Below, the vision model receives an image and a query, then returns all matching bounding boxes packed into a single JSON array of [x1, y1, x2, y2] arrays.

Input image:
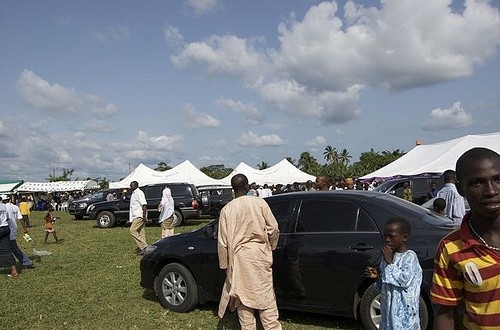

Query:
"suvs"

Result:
[[67, 188, 122, 219], [196, 186, 235, 217], [86, 183, 210, 229], [373, 175, 451, 206]]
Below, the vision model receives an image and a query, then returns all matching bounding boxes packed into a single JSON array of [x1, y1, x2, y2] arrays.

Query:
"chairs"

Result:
[[50, 202, 70, 211]]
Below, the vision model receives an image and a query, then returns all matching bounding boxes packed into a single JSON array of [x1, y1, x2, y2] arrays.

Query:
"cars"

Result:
[[139, 191, 464, 330]]
